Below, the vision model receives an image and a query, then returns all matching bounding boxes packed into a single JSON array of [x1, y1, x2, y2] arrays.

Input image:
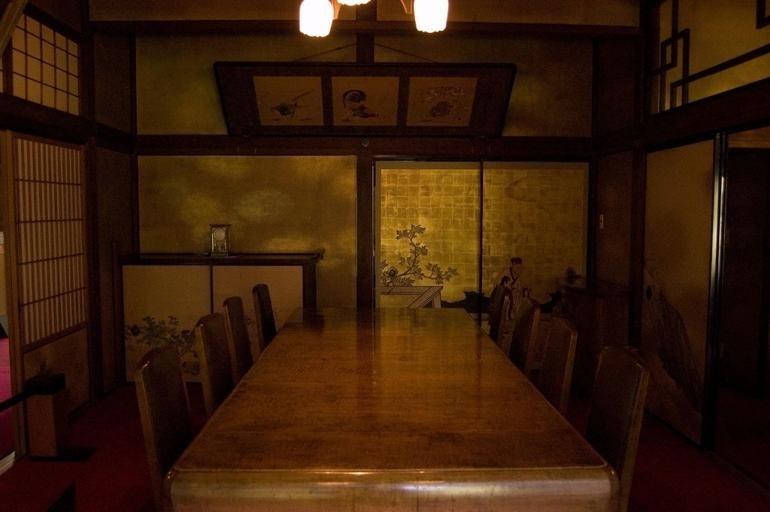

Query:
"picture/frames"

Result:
[[211, 59, 517, 139]]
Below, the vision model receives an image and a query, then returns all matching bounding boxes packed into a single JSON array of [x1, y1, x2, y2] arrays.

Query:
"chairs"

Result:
[[537, 316, 579, 416], [581, 343, 650, 511], [222, 295, 253, 388], [194, 312, 233, 423], [253, 283, 276, 353], [508, 297, 542, 379], [137, 340, 195, 512], [488, 285, 521, 360]]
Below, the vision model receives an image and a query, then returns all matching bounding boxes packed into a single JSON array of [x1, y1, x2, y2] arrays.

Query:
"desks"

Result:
[[164, 307, 622, 511]]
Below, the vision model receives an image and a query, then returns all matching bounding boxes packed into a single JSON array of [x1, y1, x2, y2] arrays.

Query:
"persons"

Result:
[[488, 257, 561, 328]]
